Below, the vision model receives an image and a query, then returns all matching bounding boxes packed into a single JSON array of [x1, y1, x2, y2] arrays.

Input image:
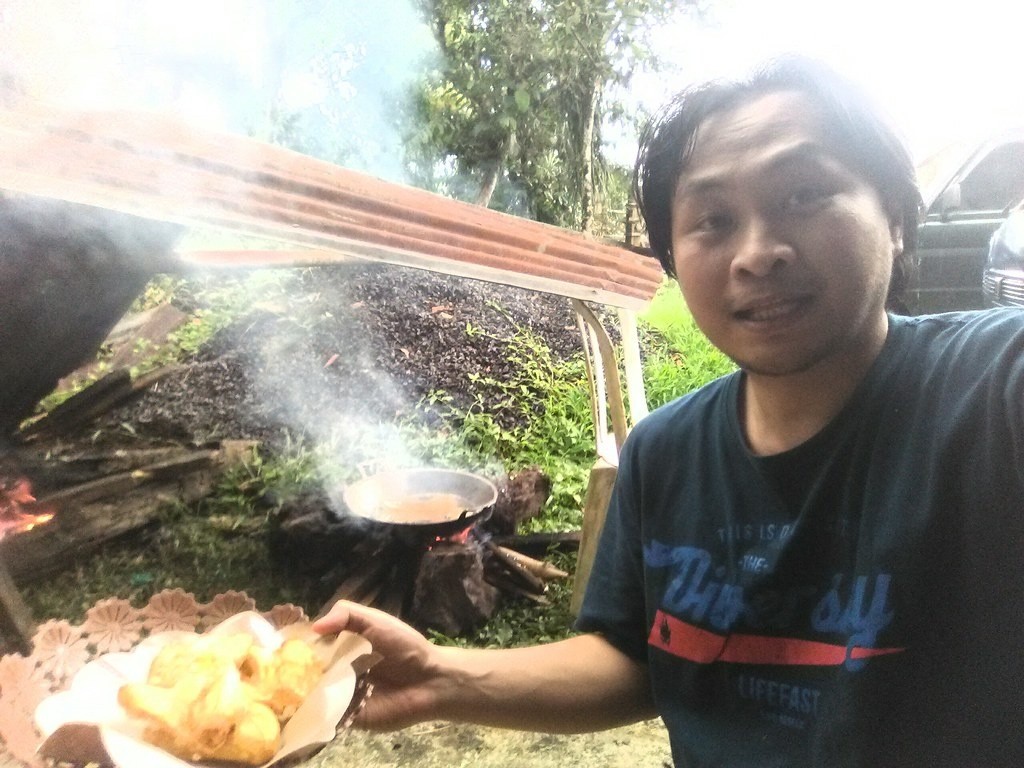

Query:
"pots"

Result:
[[344, 467, 499, 538]]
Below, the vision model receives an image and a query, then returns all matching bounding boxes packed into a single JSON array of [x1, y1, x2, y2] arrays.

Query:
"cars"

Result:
[[880, 113, 1023, 316]]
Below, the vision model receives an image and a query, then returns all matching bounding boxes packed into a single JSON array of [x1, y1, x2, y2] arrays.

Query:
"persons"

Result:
[[301, 62, 1024, 767]]
